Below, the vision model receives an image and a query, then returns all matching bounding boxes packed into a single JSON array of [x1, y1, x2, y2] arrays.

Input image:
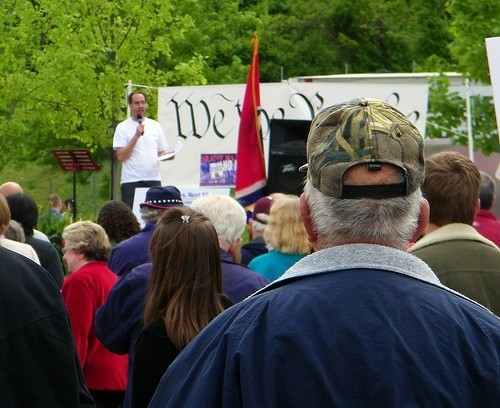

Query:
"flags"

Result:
[[234, 37, 267, 236]]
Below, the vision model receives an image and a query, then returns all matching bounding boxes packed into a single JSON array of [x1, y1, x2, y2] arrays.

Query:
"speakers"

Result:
[[267, 119, 312, 196]]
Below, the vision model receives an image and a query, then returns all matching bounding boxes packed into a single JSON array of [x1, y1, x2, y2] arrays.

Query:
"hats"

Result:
[[299, 97, 424, 199], [140, 186, 183, 209], [253, 196, 274, 225]]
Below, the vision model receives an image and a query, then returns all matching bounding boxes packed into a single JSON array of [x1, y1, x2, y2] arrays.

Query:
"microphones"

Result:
[[137, 114, 143, 136]]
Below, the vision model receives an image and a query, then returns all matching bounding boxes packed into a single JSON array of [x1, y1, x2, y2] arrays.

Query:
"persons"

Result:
[[113, 90, 176, 211], [0, 97, 500, 408]]
